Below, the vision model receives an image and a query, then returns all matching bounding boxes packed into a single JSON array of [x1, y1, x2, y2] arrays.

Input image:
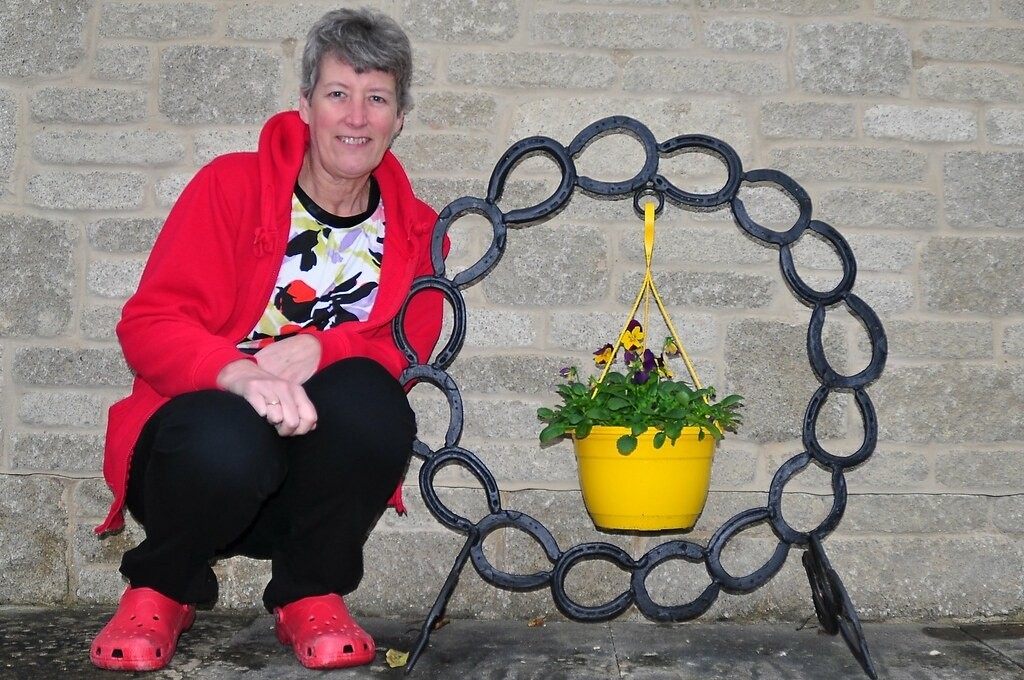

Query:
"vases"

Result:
[[564, 425, 723, 530]]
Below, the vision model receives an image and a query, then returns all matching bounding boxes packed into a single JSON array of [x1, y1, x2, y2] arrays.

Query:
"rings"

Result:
[[267, 401, 279, 406]]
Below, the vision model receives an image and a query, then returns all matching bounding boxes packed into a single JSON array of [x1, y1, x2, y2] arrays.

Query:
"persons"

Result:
[[90, 9, 449, 669]]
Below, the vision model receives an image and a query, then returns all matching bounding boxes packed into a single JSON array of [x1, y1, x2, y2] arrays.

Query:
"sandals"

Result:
[[88, 588, 197, 669], [273, 592, 376, 668]]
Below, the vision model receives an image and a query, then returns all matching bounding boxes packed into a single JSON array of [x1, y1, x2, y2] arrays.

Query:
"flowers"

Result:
[[537, 320, 745, 455]]
